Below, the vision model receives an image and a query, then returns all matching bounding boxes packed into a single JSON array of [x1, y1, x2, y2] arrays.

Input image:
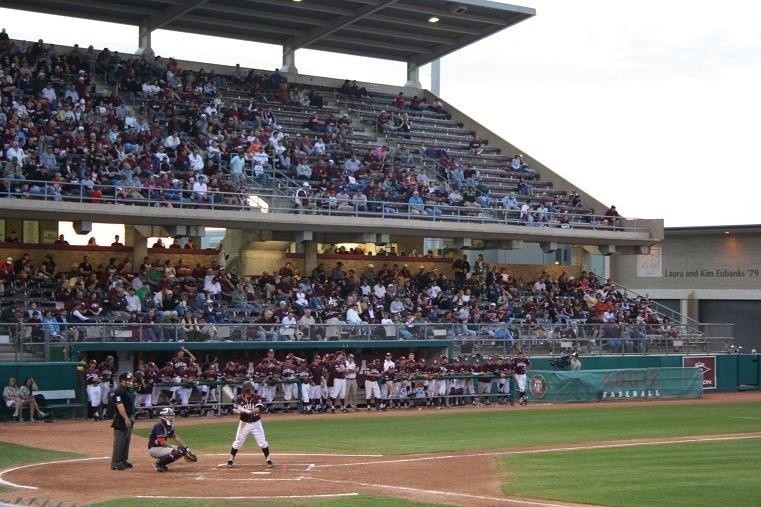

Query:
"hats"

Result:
[[571, 351, 579, 356], [147, 345, 517, 377], [89, 358, 98, 363]]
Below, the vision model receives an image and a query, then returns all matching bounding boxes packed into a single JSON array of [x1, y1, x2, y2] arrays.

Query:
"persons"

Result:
[[492, 352, 530, 405], [148, 407, 194, 474], [496, 152, 624, 232], [231, 63, 490, 222], [0, 230, 238, 342], [751, 349, 759, 355], [109, 368, 136, 470], [1, 27, 249, 211], [492, 263, 676, 353], [278, 352, 359, 414], [132, 348, 284, 415], [571, 352, 582, 370], [86, 355, 118, 420], [734, 346, 743, 355], [364, 352, 491, 411], [240, 243, 491, 341], [216, 379, 277, 469], [3, 375, 49, 423]]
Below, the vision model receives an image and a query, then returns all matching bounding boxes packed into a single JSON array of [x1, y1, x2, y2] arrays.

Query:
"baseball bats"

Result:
[[223, 385, 242, 410]]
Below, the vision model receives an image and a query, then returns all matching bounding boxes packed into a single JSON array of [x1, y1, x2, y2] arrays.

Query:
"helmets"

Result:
[[119, 370, 133, 382], [239, 380, 256, 394], [159, 407, 175, 416]]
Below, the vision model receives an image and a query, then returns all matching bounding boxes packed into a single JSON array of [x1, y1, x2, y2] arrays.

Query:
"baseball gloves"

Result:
[[184, 447, 197, 462]]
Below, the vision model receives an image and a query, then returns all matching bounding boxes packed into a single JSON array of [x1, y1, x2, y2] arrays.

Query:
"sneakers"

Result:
[[227, 454, 234, 464], [112, 461, 133, 469], [265, 454, 273, 464], [264, 393, 527, 414], [152, 458, 169, 471], [12, 410, 110, 421]]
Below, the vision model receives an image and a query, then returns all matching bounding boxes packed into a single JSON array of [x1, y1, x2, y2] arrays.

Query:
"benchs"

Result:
[[97, 70, 610, 230], [0, 272, 710, 353], [9, 389, 82, 422]]
